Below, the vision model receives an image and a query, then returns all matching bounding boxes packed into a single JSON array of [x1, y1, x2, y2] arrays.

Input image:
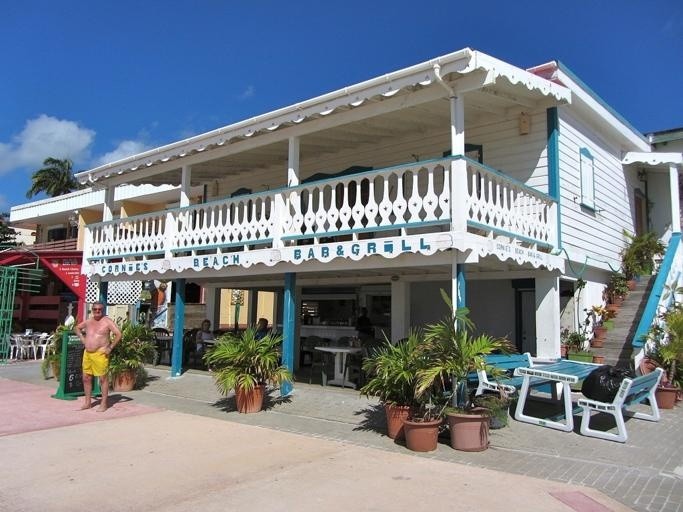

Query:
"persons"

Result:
[[254, 319, 268, 341], [196, 320, 216, 372], [74, 301, 122, 411], [303, 309, 313, 325], [355, 307, 371, 345]]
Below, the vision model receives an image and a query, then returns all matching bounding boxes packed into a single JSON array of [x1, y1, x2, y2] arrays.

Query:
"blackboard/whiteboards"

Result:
[[61, 331, 101, 397]]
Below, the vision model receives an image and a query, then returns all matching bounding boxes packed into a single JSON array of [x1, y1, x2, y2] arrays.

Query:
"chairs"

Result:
[[10, 328, 54, 362], [145, 324, 353, 369]]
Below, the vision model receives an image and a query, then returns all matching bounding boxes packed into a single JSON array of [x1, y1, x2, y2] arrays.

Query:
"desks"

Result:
[[313, 345, 363, 391], [510, 359, 605, 434]]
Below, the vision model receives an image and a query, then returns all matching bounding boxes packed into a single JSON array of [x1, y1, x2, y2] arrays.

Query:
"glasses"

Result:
[[94, 308, 101, 311]]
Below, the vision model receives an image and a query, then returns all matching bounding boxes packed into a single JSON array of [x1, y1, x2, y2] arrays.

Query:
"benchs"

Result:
[[470, 352, 559, 416], [575, 366, 665, 445]]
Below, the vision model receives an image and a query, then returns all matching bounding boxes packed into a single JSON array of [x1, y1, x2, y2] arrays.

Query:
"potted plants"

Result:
[[104, 316, 159, 393], [559, 229, 682, 410], [356, 286, 522, 453], [202, 324, 297, 415]]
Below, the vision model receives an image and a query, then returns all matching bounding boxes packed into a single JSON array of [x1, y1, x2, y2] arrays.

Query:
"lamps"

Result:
[[514, 111, 532, 136]]
